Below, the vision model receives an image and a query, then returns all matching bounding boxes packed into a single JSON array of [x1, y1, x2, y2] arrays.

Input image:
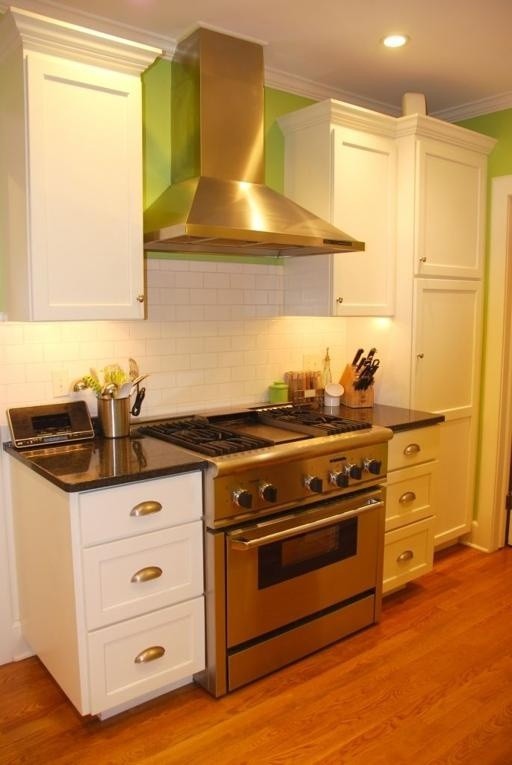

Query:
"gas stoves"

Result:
[[138, 404, 373, 457]]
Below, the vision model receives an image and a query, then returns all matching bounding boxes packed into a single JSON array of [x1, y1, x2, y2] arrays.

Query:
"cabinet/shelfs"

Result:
[[6, 455, 209, 723], [274, 96, 399, 318], [0, 5, 164, 322], [349, 112, 501, 554], [383, 423, 442, 599]]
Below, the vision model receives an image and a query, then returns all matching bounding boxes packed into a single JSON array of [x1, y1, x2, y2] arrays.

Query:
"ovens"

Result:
[[212, 443, 388, 694]]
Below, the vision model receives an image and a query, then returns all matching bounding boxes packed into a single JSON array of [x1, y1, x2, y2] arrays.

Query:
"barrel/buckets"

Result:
[[96, 394, 131, 438]]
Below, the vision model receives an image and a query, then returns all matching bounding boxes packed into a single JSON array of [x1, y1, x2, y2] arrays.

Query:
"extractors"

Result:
[[144, 27, 366, 259]]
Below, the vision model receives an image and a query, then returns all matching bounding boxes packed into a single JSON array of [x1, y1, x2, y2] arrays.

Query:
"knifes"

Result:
[[352, 348, 380, 391]]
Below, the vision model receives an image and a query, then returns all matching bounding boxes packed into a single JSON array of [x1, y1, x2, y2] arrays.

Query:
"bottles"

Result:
[[322, 347, 333, 388], [269, 379, 289, 404]]
